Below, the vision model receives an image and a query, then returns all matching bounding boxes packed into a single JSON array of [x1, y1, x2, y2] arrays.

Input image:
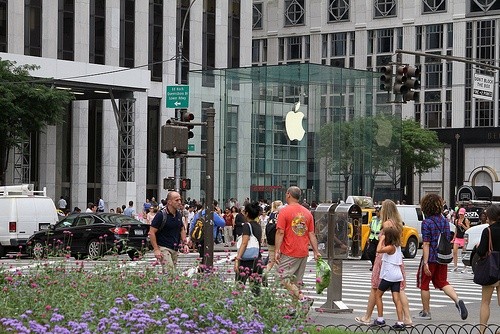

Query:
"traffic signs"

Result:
[[166, 85, 190, 109]]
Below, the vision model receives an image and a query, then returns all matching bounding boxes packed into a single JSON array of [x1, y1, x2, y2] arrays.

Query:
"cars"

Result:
[[27, 212, 151, 261], [461, 223, 490, 266], [348, 208, 420, 260]]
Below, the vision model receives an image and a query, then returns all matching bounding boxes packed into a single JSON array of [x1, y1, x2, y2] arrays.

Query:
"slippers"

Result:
[[355, 317, 372, 325], [404, 323, 414, 328]]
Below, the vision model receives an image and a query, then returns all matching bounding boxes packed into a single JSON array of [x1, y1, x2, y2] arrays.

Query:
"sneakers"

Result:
[[373, 319, 385, 327], [390, 322, 404, 331], [415, 310, 431, 320], [455, 299, 468, 320]]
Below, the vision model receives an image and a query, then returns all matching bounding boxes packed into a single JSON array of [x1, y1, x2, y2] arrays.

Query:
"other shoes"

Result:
[[461, 266, 469, 273], [286, 308, 296, 315], [370, 266, 373, 271], [299, 297, 314, 319], [450, 267, 458, 272]]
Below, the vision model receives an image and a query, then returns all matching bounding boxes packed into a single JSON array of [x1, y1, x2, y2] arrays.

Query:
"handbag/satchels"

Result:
[[237, 222, 259, 261], [474, 227, 500, 285], [316, 256, 332, 294]]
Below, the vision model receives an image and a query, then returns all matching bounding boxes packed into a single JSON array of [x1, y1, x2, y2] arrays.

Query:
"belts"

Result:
[[371, 231, 379, 234]]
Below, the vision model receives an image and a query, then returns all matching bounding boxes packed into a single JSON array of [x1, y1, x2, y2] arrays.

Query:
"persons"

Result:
[[354, 199, 415, 329], [149, 191, 190, 283], [273, 186, 322, 320], [233, 202, 263, 299], [412, 194, 470, 320], [470, 205, 500, 334], [56, 195, 500, 332], [370, 227, 407, 331]]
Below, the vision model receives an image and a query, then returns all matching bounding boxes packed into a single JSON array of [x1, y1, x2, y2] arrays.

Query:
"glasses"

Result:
[[375, 211, 379, 213]]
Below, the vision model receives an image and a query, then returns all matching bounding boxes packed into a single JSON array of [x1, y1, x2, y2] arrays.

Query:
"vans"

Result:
[[376, 205, 457, 249], [0, 184, 59, 257]]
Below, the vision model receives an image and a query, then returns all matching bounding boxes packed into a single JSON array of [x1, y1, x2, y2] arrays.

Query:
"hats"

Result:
[[374, 205, 382, 211], [459, 208, 465, 215]]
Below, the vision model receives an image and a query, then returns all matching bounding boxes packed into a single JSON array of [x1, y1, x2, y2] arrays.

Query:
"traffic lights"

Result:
[[380, 65, 392, 90], [404, 65, 420, 103], [180, 178, 191, 191], [164, 177, 174, 191], [395, 63, 409, 95], [181, 109, 195, 139]]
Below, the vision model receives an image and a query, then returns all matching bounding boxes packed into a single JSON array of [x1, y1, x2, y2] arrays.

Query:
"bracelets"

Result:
[[235, 256, 240, 261], [182, 244, 189, 249], [423, 262, 428, 265]]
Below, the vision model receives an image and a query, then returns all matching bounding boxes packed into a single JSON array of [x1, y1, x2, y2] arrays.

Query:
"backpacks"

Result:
[[266, 218, 276, 245], [190, 211, 204, 244], [430, 218, 453, 265]]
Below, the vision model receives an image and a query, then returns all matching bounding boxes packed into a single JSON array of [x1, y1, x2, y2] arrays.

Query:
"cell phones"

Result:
[[214, 207, 218, 211]]
